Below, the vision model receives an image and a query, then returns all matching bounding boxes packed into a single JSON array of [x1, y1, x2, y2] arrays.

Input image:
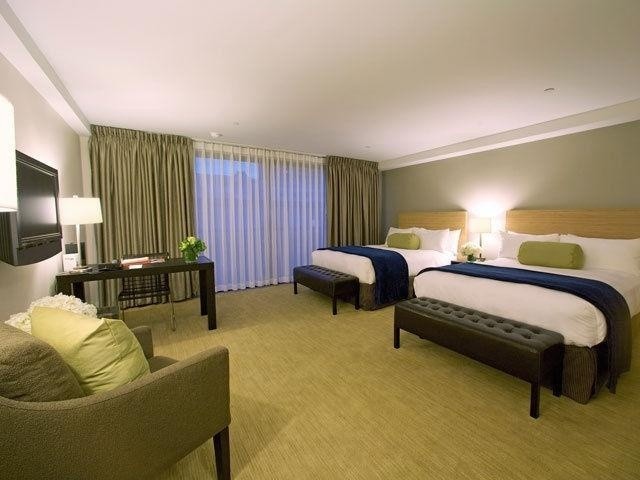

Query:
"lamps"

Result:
[[57, 196, 105, 274], [470, 218, 492, 262], [0, 95, 20, 213]]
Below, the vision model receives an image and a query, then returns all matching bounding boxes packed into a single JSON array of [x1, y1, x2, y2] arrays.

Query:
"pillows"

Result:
[[384, 226, 462, 253], [496, 229, 559, 259], [31, 299, 153, 396], [0, 319, 84, 402], [560, 233, 639, 275], [517, 240, 583, 270]]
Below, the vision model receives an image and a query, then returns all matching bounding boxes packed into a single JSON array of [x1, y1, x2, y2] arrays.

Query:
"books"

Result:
[[121, 252, 167, 269]]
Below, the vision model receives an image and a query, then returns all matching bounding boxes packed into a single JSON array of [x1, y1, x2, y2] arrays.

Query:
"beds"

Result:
[[412, 208, 640, 403], [311, 210, 468, 311]]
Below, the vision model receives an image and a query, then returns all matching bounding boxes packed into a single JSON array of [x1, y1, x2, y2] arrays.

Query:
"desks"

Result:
[[55, 254, 215, 333]]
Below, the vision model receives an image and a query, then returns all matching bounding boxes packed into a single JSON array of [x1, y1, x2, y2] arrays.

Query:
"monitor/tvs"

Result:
[[0, 149, 62, 266]]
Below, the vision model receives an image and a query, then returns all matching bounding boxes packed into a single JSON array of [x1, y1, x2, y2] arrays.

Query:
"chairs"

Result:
[[0, 322, 231, 480], [116, 251, 178, 332]]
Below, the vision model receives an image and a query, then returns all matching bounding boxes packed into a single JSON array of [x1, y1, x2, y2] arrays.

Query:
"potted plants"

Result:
[[178, 234, 206, 265]]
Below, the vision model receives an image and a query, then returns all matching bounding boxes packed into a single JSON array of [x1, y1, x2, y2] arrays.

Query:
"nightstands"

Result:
[[451, 258, 468, 266]]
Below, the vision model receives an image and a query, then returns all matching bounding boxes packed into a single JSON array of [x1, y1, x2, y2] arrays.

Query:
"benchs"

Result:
[[293, 266, 362, 315], [394, 297, 564, 419]]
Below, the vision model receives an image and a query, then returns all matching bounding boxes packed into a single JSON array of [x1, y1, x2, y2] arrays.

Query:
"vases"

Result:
[[467, 255, 475, 261]]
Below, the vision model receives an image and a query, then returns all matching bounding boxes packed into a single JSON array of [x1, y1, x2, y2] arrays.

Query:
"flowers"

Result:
[[462, 241, 483, 257]]
